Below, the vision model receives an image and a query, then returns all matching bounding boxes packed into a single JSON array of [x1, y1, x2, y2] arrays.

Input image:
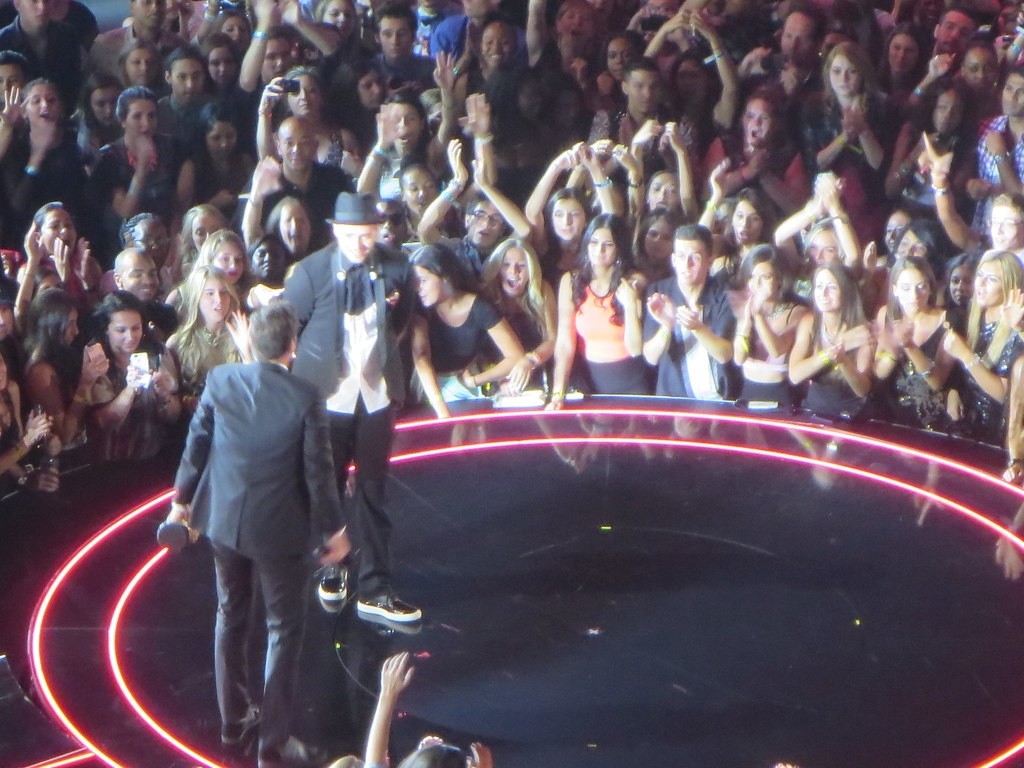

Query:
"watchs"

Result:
[[16, 473, 31, 487], [1009, 458, 1021, 466]]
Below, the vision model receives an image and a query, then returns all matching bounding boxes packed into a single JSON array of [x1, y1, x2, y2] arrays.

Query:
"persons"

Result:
[[282, 194, 421, 621], [165, 306, 351, 768], [1, 0, 1024, 491], [329, 652, 492, 768], [451, 413, 1024, 580]]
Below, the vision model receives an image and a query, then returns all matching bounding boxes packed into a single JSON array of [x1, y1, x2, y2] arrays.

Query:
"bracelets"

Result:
[[965, 354, 980, 369], [452, 67, 460, 77], [820, 351, 832, 366], [205, 12, 217, 22], [932, 184, 950, 193], [450, 180, 464, 193], [594, 177, 611, 187], [249, 198, 262, 207], [736, 333, 750, 339], [367, 156, 380, 167], [628, 178, 643, 188], [883, 352, 897, 363], [254, 31, 270, 40], [74, 394, 90, 404], [921, 361, 937, 377], [994, 152, 1011, 163], [259, 110, 273, 118], [170, 390, 178, 396], [475, 133, 494, 145], [442, 190, 461, 209], [707, 202, 719, 211], [373, 148, 388, 158]]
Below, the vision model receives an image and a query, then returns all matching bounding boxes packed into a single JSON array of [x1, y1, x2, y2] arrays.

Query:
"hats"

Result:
[[325, 191, 380, 226]]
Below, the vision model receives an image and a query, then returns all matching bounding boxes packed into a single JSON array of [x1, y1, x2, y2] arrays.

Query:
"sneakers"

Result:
[[222, 704, 262, 743], [257, 736, 329, 768]]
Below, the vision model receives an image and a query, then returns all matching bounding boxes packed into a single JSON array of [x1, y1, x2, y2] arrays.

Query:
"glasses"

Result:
[[469, 210, 504, 226], [379, 212, 405, 224], [133, 237, 170, 248]]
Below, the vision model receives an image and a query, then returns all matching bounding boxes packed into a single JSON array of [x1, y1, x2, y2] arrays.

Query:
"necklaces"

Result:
[[762, 301, 787, 319], [822, 325, 832, 345]]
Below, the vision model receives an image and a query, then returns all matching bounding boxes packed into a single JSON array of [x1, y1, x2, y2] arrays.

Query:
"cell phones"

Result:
[[692, 28, 703, 43], [275, 80, 300, 93], [131, 352, 150, 389], [88, 343, 106, 365]]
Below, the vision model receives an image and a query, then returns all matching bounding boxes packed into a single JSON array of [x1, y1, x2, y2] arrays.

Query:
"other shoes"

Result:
[[357, 592, 422, 622], [319, 565, 347, 600]]
